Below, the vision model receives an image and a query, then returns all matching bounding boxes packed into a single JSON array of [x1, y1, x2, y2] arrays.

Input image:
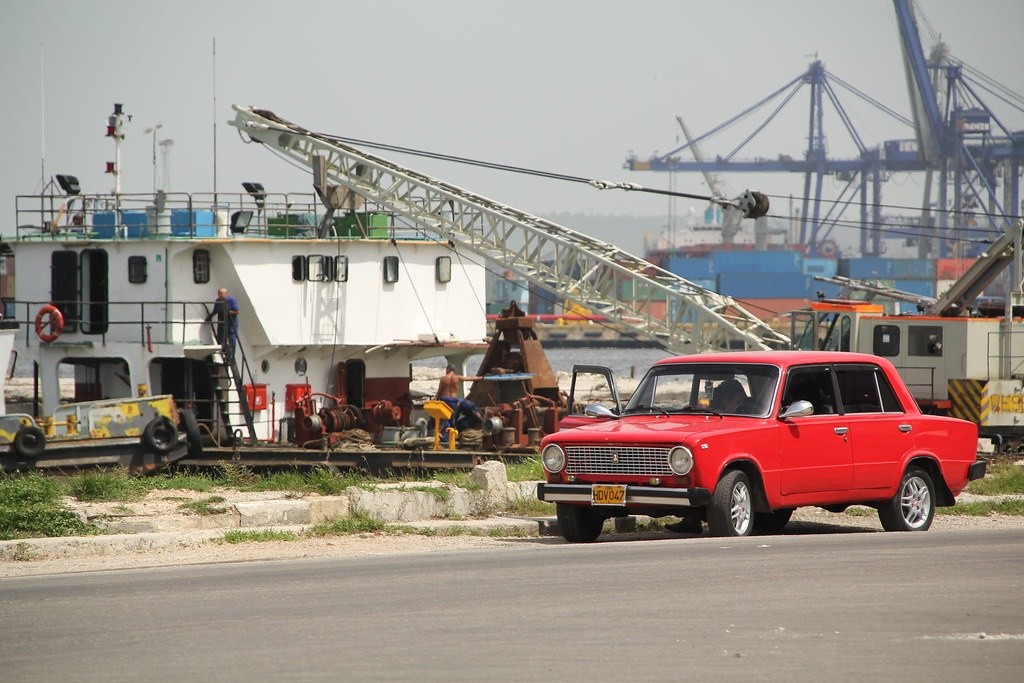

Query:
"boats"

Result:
[[0, 104, 589, 477], [789, 218, 1024, 438]]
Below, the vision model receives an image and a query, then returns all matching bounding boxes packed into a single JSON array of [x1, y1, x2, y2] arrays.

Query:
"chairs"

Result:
[[783, 380, 832, 415], [712, 387, 742, 415]]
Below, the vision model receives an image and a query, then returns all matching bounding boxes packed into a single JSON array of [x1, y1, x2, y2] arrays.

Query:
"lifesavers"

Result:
[[141, 415, 178, 454], [179, 409, 204, 458], [34, 305, 66, 344], [12, 425, 47, 457]]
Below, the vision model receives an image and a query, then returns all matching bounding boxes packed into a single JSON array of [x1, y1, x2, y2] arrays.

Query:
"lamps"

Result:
[[230, 211, 253, 236], [242, 182, 267, 235], [56, 174, 82, 233]]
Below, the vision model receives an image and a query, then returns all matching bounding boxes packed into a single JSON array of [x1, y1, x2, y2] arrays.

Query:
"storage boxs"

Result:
[[330, 213, 389, 239], [171, 210, 218, 236], [286, 384, 311, 410], [268, 215, 297, 235], [93, 212, 147, 237], [240, 383, 268, 411]]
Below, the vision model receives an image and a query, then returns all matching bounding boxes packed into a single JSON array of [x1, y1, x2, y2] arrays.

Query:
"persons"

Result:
[[204, 288, 240, 361], [436, 364, 483, 441], [714, 380, 768, 415]]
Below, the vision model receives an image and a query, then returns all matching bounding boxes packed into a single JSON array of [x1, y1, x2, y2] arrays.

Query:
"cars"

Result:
[[537, 350, 987, 544]]
